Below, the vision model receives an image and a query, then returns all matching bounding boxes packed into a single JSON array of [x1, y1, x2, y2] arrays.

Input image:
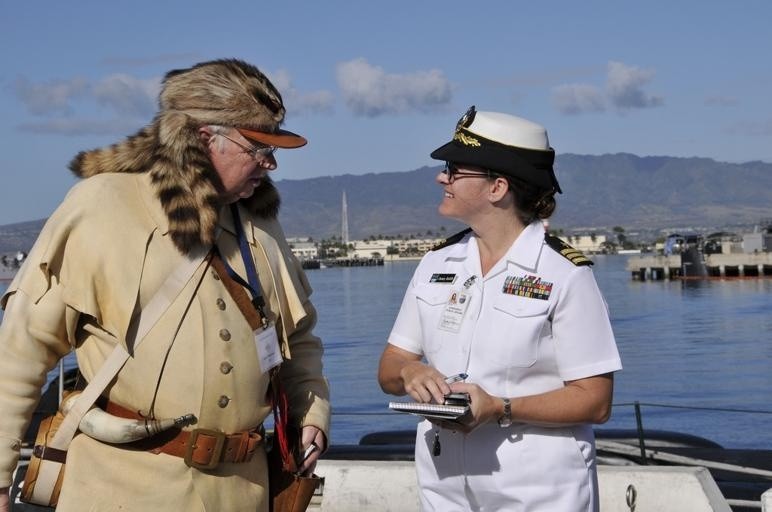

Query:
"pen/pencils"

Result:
[[445, 372, 469, 385]]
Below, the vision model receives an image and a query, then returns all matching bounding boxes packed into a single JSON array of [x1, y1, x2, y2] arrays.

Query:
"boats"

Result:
[[296, 401, 771, 511]]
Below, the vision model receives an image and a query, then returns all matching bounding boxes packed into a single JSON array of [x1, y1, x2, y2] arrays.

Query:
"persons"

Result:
[[0, 58, 331, 511], [377, 105, 622, 512]]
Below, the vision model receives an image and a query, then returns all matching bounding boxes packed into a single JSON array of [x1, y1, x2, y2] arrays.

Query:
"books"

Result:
[[388, 401, 468, 424]]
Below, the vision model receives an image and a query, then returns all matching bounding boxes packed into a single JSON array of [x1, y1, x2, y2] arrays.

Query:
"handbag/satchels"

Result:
[[266, 432, 321, 512], [19, 410, 77, 509]]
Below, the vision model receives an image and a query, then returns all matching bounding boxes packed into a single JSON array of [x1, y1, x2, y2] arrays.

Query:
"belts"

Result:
[[92, 395, 266, 472]]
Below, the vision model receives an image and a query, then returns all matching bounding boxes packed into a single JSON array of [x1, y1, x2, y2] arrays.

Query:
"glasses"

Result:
[[214, 129, 279, 161], [444, 161, 501, 181]]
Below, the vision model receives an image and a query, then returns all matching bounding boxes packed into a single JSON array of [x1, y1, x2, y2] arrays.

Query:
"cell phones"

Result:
[[445, 392, 468, 406]]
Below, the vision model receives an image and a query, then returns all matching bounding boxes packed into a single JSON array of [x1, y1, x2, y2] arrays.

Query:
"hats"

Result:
[[160, 57, 308, 151], [430, 105, 563, 195]]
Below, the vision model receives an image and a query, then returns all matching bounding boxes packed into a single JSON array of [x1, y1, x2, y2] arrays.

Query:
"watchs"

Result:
[[499, 397, 513, 429]]
